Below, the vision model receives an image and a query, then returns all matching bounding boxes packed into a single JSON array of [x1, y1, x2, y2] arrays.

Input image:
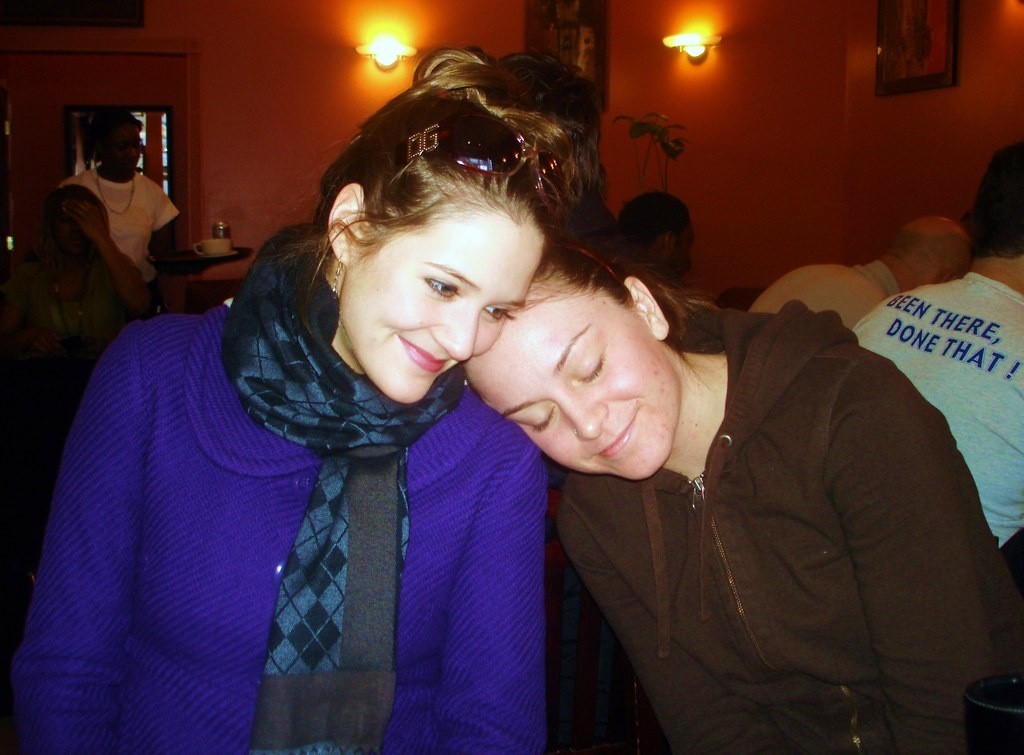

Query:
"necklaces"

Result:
[[95, 174, 134, 214], [53, 270, 83, 337]]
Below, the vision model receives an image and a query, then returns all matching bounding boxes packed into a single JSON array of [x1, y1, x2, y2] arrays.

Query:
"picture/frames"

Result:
[[875, 0, 959, 95], [0, 0, 144, 28]]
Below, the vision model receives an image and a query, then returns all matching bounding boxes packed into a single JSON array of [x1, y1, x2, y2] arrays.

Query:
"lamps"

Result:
[[356, 43, 417, 69], [663, 32, 721, 62]]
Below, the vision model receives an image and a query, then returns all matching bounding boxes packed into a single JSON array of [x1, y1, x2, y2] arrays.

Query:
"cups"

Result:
[[964, 674, 1024, 755], [193, 239, 231, 255]]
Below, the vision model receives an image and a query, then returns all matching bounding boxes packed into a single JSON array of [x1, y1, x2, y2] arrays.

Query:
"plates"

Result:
[[197, 250, 238, 257]]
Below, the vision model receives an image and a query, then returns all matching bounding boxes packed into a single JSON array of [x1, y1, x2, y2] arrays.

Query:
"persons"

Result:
[[621, 192, 693, 277], [465, 207, 1024, 754], [13, 46, 606, 755], [0, 185, 150, 354], [749, 217, 971, 329], [57, 108, 179, 322], [849, 142, 1024, 546]]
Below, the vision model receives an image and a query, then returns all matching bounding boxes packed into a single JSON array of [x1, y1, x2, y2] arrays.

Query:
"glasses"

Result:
[[394, 111, 571, 220]]
